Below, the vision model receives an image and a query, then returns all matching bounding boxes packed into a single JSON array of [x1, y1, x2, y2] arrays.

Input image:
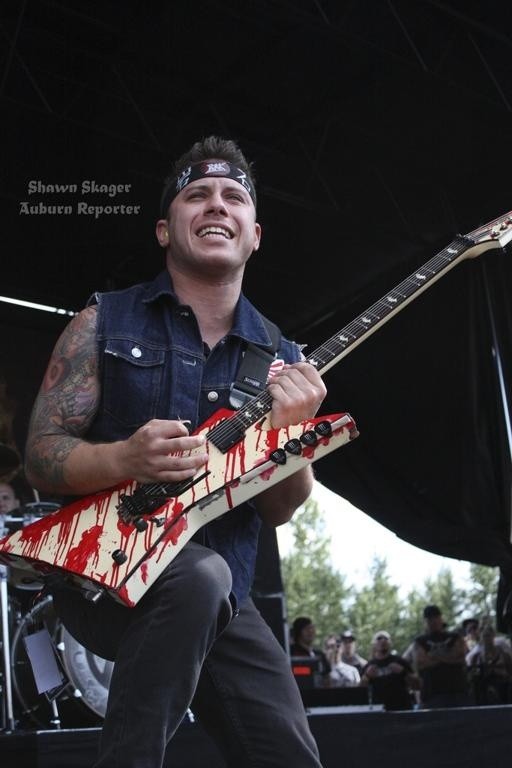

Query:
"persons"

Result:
[[22, 133, 331, 768], [290, 607, 512, 709], [0, 481, 20, 516]]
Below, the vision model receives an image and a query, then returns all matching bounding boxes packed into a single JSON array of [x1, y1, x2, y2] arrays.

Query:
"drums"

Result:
[[12, 595, 114, 728], [0, 515, 45, 591]]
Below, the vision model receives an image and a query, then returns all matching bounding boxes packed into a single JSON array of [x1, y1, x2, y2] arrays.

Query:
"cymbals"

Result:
[[5, 502, 61, 517]]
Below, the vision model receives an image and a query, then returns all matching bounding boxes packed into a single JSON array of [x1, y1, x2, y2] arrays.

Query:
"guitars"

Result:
[[0, 214, 512, 613]]
